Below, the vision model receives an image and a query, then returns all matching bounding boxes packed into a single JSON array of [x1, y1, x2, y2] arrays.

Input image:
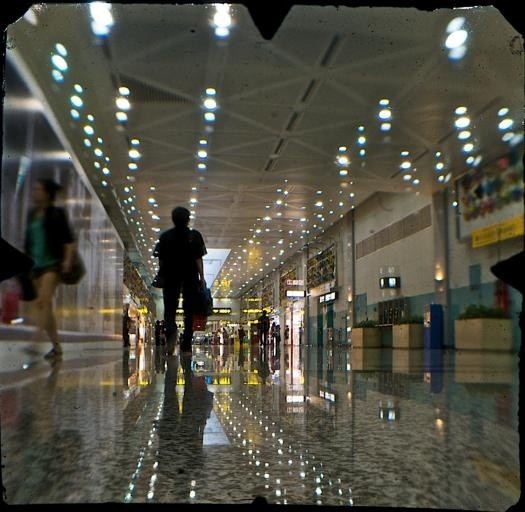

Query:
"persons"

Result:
[[150, 207, 208, 353], [123, 308, 289, 347], [20, 176, 78, 361]]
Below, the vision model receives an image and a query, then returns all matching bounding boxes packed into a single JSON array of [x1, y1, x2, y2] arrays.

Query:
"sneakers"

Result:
[[43, 348, 64, 361], [163, 327, 193, 354]]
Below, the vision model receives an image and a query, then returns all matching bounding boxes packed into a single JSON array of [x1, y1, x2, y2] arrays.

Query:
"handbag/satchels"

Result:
[[59, 258, 88, 286], [197, 279, 213, 317], [151, 274, 166, 289]]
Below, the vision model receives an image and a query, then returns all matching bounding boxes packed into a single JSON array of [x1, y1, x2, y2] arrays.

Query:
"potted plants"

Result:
[[454, 304, 514, 353], [392, 314, 424, 349], [351, 320, 382, 348]]
